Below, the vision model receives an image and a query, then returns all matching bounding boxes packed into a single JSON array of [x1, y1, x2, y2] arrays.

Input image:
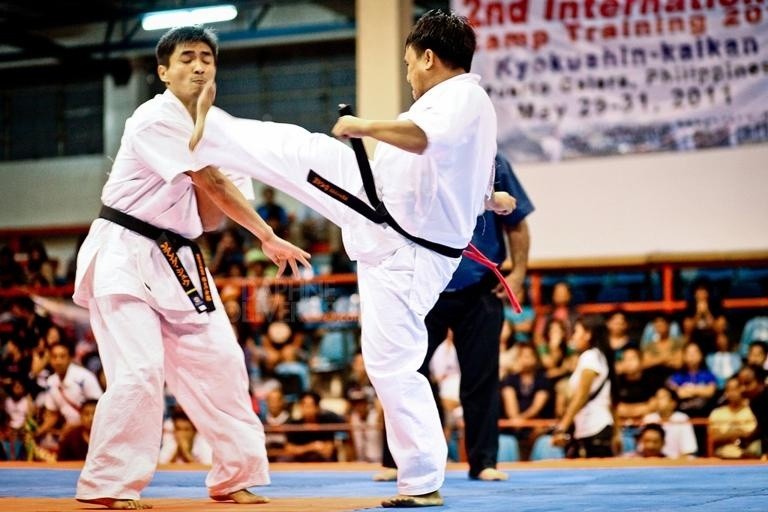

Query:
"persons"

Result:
[[156, 184, 380, 464], [0, 233, 107, 461], [430, 274, 768, 459], [367, 151, 538, 484], [72, 30, 312, 512], [183, 5, 500, 506]]
